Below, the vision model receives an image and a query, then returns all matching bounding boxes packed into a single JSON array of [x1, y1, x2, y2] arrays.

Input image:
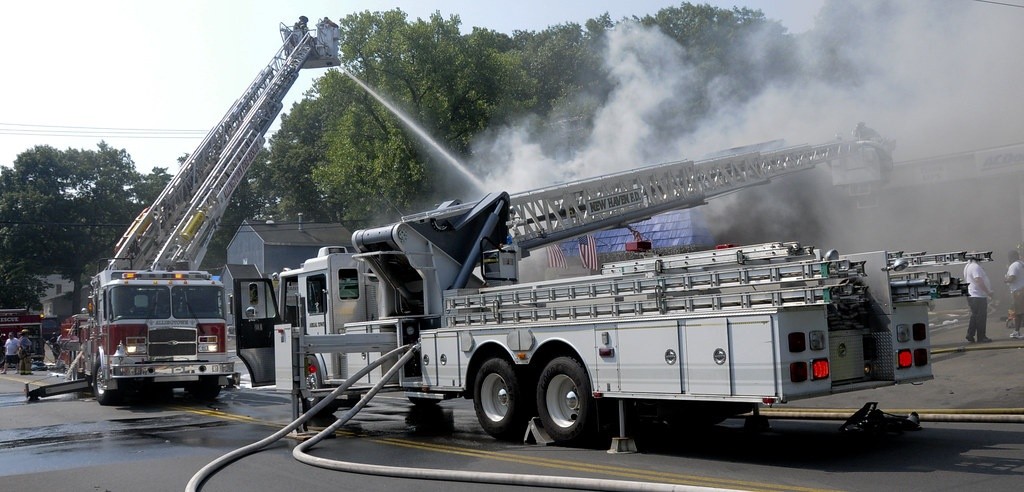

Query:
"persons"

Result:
[[1004, 250, 1024, 340], [0, 329, 34, 375], [50, 337, 61, 364], [965, 251, 994, 343]]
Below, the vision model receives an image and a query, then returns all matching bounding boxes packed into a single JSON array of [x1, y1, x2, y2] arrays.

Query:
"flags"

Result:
[[578, 234, 598, 271], [546, 244, 568, 270]]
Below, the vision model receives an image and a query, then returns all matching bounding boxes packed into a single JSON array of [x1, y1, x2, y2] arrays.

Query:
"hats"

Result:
[[19, 329, 29, 335], [9, 332, 14, 336]]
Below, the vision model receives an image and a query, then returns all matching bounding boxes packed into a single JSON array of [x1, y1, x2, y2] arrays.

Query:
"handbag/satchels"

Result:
[[18, 346, 26, 359]]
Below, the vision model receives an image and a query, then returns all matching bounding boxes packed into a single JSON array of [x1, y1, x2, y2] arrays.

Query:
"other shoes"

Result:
[[25, 371, 33, 374], [977, 335, 992, 342], [965, 333, 975, 342], [1, 371, 6, 373], [15, 370, 18, 373], [20, 371, 25, 375]]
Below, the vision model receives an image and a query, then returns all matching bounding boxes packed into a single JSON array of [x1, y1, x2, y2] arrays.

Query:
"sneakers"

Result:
[[1010, 331, 1019, 337]]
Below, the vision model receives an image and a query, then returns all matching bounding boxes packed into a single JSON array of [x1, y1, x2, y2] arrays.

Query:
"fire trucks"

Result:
[[0, 308, 44, 370], [23, 16, 342, 408], [230, 119, 995, 455]]
